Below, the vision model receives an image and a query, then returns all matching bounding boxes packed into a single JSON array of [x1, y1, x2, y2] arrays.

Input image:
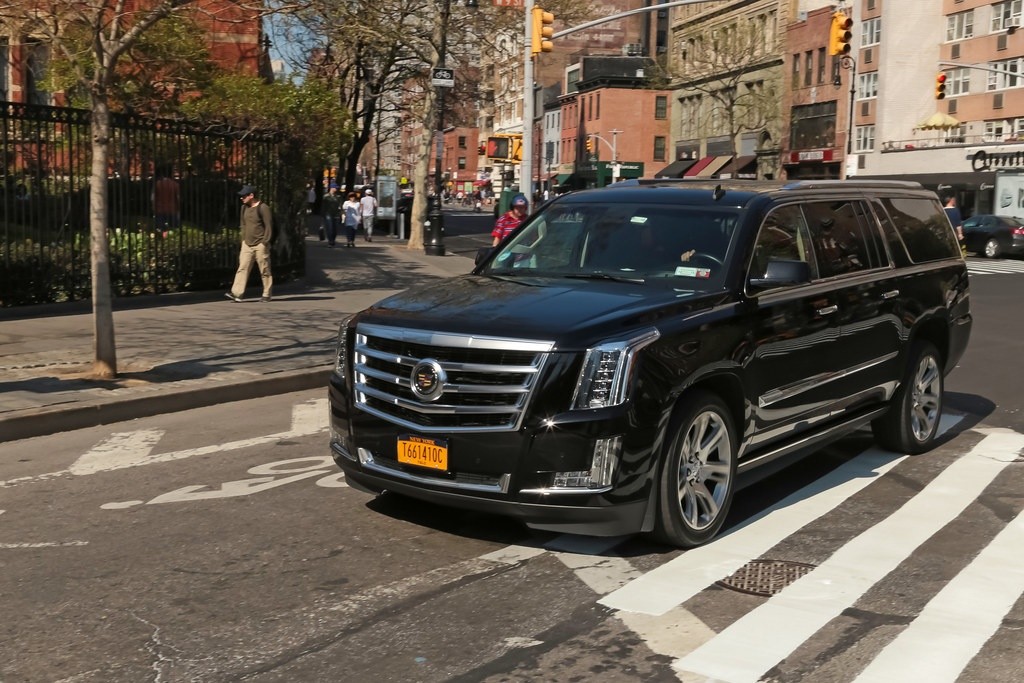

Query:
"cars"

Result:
[[959, 214, 1024, 259]]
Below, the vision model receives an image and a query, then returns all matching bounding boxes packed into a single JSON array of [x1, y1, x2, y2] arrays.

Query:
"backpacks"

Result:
[[243, 203, 279, 244]]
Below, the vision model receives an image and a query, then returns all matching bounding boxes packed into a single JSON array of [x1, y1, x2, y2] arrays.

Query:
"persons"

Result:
[[224, 185, 273, 302], [151, 170, 182, 226], [305, 183, 378, 247], [534, 188, 565, 202], [491, 195, 531, 270], [943, 195, 964, 250], [443, 189, 494, 207]]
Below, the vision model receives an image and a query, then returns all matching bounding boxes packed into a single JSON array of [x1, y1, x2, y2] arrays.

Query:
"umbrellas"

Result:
[[913, 110, 962, 146]]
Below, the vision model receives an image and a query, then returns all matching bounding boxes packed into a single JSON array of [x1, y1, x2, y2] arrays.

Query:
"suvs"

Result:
[[327, 180, 973, 550]]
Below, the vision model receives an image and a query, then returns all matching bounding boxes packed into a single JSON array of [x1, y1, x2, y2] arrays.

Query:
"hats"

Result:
[[365, 189, 373, 195], [237, 186, 253, 196], [512, 195, 527, 207]]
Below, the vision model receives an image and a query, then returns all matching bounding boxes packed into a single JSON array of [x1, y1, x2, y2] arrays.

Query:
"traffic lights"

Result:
[[487, 137, 509, 158], [828, 16, 854, 55], [531, 8, 554, 53], [935, 73, 946, 99], [585, 140, 591, 151]]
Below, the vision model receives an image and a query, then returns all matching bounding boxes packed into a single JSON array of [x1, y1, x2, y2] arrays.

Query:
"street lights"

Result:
[[833, 56, 857, 154], [423, 1, 480, 256]]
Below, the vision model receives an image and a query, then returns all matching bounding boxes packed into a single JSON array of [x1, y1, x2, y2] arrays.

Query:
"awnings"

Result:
[[715, 155, 757, 180], [532, 172, 557, 181], [550, 174, 573, 188], [683, 155, 733, 179], [655, 160, 698, 179]]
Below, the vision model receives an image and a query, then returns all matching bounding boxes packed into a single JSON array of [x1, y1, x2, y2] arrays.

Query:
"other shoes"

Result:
[[364, 237, 372, 242], [259, 297, 270, 302], [225, 293, 238, 301], [348, 242, 354, 247], [328, 243, 333, 248]]
